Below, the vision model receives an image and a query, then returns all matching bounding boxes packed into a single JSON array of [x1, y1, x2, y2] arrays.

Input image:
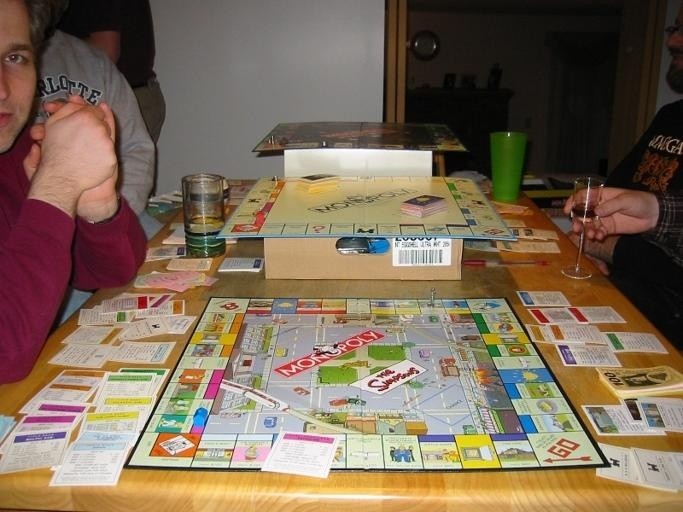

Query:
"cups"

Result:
[[181, 173, 226, 258], [490, 132, 529, 202]]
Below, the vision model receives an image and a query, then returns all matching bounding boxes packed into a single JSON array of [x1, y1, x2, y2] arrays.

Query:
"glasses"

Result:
[[663, 23, 682, 34]]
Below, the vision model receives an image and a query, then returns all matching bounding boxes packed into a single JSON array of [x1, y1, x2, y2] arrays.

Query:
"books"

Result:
[[296, 174, 337, 195], [400, 194, 447, 218], [596, 365, 683, 398]]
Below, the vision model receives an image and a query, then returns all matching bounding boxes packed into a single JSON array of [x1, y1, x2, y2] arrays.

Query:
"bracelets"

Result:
[[87, 192, 121, 224], [27, 196, 73, 219]]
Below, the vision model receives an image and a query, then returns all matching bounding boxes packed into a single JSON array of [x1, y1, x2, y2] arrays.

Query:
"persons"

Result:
[[45, 1, 167, 144], [567, 0, 683, 351], [562, 187, 683, 268], [0, 1, 147, 384], [26, 29, 163, 334]]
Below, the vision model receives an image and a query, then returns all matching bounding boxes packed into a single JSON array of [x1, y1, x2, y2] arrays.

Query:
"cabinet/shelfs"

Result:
[[405, 86, 514, 173]]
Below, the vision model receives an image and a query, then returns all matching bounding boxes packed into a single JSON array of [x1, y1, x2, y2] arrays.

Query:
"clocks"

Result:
[[411, 29, 441, 61]]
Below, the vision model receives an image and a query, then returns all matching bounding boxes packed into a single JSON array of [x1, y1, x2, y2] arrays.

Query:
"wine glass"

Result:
[[560, 176, 604, 280]]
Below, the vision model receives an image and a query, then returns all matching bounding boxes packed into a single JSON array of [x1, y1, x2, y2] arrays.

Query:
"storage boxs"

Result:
[[263, 236, 464, 281], [283, 147, 434, 178]]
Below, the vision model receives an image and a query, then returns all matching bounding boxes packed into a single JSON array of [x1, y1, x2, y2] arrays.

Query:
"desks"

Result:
[[1, 177, 682, 511], [253, 122, 469, 177]]
